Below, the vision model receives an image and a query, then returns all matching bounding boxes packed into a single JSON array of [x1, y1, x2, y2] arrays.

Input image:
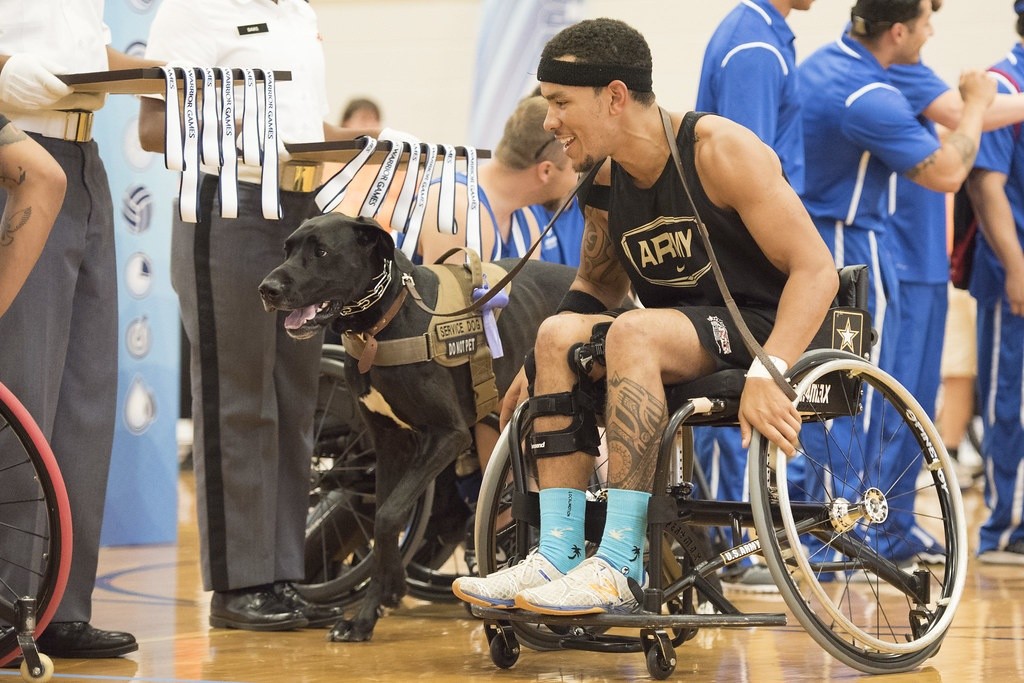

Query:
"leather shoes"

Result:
[[209, 581, 344, 630], [36, 621, 138, 657], [0, 626, 39, 666]]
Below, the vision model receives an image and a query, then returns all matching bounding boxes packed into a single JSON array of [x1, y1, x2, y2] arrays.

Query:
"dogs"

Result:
[[257, 213, 639, 643]]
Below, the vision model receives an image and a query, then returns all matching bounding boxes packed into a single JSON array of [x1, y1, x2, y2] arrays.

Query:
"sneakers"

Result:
[[452, 547, 567, 609], [514, 557, 649, 615]]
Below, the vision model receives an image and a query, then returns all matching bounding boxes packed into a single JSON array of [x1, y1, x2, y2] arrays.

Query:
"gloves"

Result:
[[377, 127, 422, 144], [237, 125, 293, 165], [0, 55, 74, 111], [160, 61, 210, 107]]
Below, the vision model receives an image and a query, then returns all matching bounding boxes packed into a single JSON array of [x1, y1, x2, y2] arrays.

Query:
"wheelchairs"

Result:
[[0, 382, 73, 683], [469, 261, 969, 679], [287, 340, 507, 603]]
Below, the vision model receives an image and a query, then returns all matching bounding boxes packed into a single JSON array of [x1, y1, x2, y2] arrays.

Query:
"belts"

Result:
[[0, 108, 95, 142], [200, 159, 324, 193]]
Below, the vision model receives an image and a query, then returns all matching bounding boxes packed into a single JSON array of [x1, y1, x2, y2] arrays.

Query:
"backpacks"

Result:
[[949, 68, 1022, 290]]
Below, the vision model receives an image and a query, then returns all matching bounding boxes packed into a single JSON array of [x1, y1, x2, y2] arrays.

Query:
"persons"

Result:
[[0, 0, 173, 670], [453, 20, 841, 615], [343, 0, 1024, 596], [0, 114, 67, 318], [138, 0, 387, 633]]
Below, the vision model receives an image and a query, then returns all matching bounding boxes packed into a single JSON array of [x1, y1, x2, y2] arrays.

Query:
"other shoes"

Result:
[[834, 559, 919, 583], [720, 562, 799, 593], [978, 540, 1024, 564]]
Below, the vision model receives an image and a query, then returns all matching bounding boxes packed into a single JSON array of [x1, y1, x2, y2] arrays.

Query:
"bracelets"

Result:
[[748, 355, 788, 380]]
